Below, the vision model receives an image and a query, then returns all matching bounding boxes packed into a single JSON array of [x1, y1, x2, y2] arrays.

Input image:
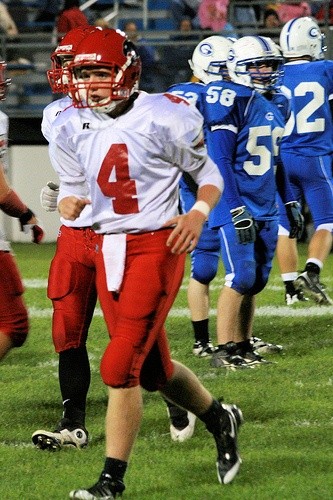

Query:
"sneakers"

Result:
[[193, 338, 216, 357], [68, 470, 125, 500], [210, 342, 278, 372], [293, 271, 333, 305], [31, 417, 89, 451], [249, 335, 280, 354], [162, 395, 197, 442], [206, 397, 245, 485], [285, 289, 309, 305]]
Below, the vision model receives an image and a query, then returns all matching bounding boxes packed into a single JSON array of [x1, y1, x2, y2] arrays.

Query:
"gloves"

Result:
[[19, 208, 45, 245], [231, 208, 259, 245], [286, 202, 305, 239], [40, 180, 59, 212]]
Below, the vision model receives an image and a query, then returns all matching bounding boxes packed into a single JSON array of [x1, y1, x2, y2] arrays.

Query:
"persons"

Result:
[[0, 55, 44, 361], [31, 25, 245, 500], [168, 17, 333, 369], [0, 0, 333, 95], [188, 200, 210, 220]]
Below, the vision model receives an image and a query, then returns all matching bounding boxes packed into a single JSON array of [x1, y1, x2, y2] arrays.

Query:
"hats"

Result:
[[263, 9, 279, 24]]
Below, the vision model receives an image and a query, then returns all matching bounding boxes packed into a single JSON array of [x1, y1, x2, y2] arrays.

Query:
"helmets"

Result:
[[73, 27, 142, 99], [0, 57, 10, 101], [279, 16, 324, 62], [188, 34, 287, 95], [53, 24, 100, 85]]
